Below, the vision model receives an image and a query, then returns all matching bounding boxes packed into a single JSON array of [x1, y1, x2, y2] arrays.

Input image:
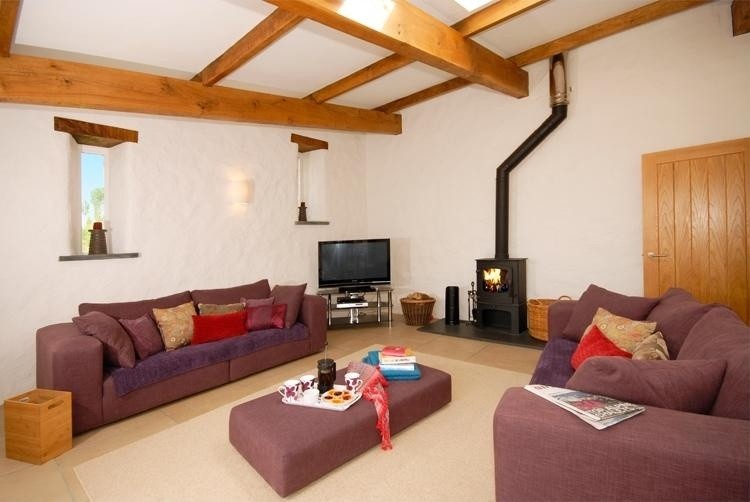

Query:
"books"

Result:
[[379, 364, 414, 372], [379, 348, 416, 363]]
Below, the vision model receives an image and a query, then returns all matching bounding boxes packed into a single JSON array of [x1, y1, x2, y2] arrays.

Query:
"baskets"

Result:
[[400, 297, 436, 325], [528, 296, 571, 341]]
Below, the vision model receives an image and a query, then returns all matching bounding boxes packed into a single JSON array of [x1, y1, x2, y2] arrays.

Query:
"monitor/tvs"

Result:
[[318, 239, 391, 292]]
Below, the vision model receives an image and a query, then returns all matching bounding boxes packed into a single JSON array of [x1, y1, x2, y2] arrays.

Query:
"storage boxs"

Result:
[[3, 387, 75, 466]]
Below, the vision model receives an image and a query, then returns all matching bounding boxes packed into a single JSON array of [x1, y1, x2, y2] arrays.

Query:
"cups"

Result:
[[300, 375, 316, 392], [277, 379, 302, 403], [302, 388, 319, 405], [317, 358, 336, 397], [344, 372, 364, 393]]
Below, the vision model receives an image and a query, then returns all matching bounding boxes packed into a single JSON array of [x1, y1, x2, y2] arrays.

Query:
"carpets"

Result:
[[70, 339, 535, 501]]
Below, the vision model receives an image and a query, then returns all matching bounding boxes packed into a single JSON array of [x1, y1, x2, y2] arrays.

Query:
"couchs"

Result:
[[491, 288, 749, 502], [33, 276, 328, 441]]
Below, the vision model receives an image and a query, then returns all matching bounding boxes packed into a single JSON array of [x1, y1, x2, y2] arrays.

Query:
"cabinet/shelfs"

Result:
[[315, 285, 394, 331]]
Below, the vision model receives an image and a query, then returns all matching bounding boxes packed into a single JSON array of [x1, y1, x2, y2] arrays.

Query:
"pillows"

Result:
[[561, 282, 730, 415], [190, 282, 308, 346], [71, 310, 136, 371], [116, 311, 165, 360], [150, 300, 198, 353]]
